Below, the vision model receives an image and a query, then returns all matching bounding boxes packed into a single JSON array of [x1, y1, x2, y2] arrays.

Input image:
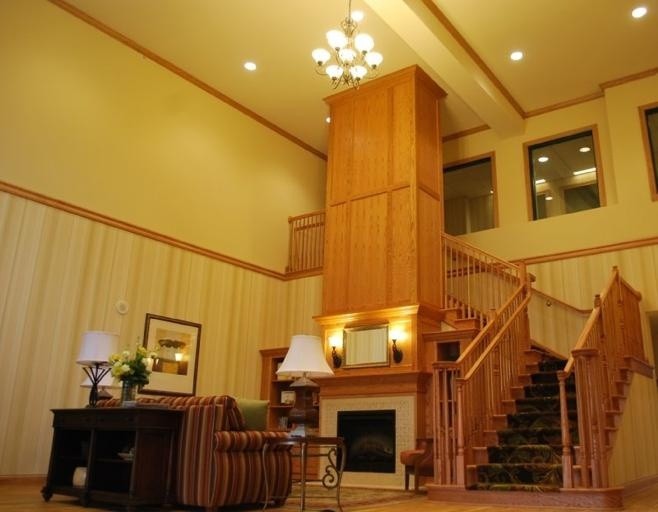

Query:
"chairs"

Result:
[[399, 437, 434, 496]]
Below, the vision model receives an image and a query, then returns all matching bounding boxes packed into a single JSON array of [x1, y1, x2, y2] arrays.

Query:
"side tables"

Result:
[[259, 436, 347, 512]]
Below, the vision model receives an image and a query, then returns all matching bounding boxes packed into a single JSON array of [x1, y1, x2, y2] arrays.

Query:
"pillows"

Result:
[[234, 397, 271, 431]]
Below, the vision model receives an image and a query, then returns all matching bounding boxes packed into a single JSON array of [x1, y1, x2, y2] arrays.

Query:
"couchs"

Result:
[[89, 395, 294, 512]]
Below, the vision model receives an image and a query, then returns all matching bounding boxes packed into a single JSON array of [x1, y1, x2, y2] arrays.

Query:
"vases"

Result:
[[120, 379, 140, 408]]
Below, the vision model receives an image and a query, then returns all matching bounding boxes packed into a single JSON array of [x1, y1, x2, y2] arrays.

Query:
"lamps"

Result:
[[311, 0, 384, 92], [275, 335, 335, 435], [73, 331, 123, 406]]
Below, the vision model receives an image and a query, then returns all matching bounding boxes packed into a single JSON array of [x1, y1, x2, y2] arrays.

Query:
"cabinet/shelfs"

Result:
[[258, 347, 320, 482], [40, 407, 184, 512]]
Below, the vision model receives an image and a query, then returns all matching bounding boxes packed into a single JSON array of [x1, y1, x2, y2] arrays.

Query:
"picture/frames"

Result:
[[137, 312, 203, 399], [341, 321, 392, 370]]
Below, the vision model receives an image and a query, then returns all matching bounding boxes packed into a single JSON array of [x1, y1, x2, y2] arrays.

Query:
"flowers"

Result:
[[108, 344, 158, 400]]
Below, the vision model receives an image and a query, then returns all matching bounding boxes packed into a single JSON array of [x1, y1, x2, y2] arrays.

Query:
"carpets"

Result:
[[250, 481, 426, 511]]
